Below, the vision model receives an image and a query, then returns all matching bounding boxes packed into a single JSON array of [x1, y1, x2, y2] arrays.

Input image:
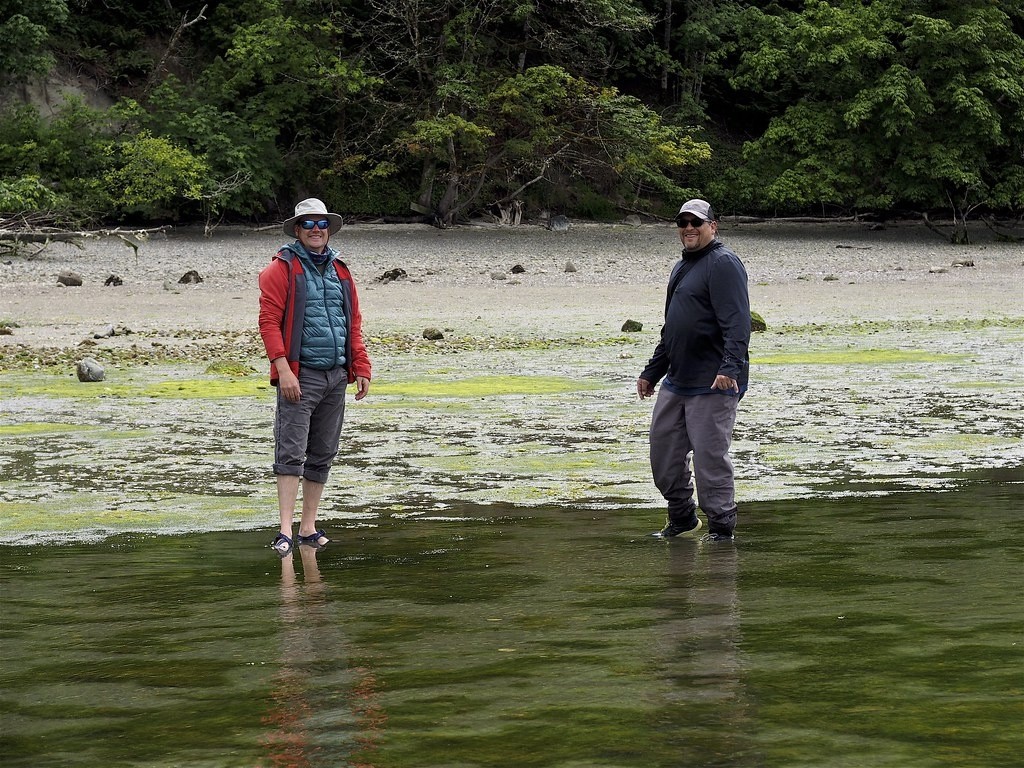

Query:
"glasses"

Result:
[[299, 219, 330, 230], [675, 217, 710, 228]]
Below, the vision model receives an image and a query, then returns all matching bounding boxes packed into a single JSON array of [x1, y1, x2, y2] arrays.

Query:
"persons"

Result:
[[258, 196, 371, 553], [637, 198, 751, 544]]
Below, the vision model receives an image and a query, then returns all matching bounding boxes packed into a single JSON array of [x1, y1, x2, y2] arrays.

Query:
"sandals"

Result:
[[274, 533, 293, 552], [297, 528, 330, 547]]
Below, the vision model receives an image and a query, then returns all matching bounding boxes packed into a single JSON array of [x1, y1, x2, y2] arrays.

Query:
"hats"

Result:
[[283, 198, 343, 239], [676, 199, 714, 222]]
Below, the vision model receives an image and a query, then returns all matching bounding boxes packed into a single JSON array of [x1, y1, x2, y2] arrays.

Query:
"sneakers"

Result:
[[660, 517, 702, 537], [699, 532, 734, 541]]
